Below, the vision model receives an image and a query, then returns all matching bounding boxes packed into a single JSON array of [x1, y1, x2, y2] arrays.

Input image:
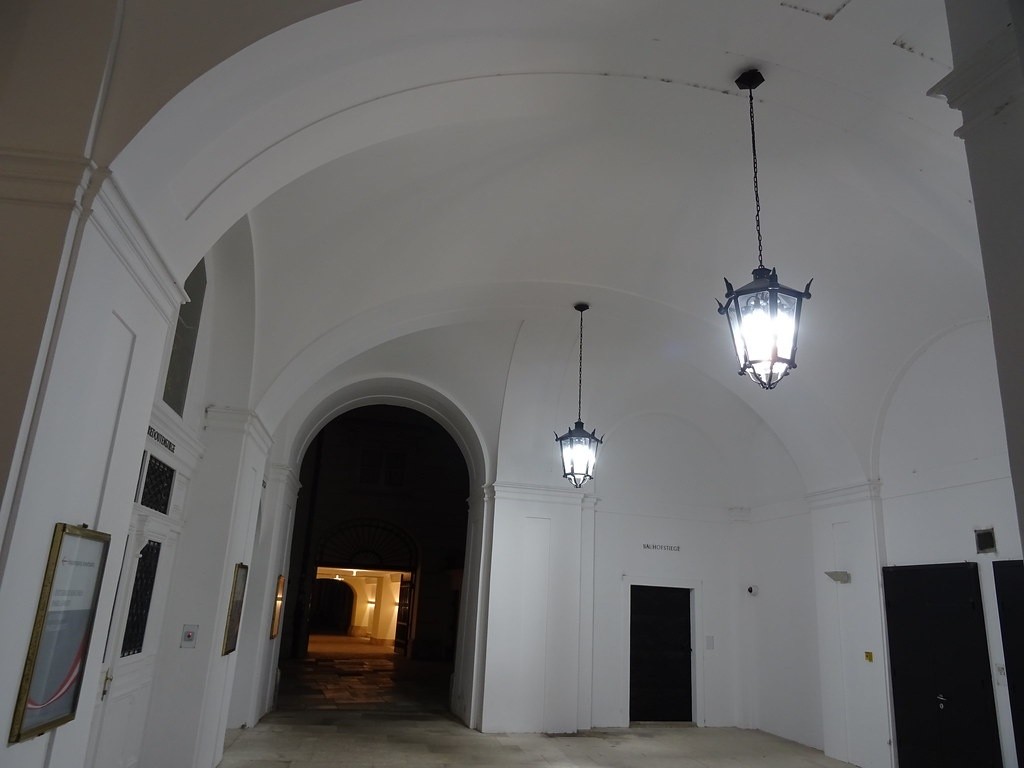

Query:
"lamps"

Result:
[[715, 69, 813, 390], [552, 303, 605, 488]]
